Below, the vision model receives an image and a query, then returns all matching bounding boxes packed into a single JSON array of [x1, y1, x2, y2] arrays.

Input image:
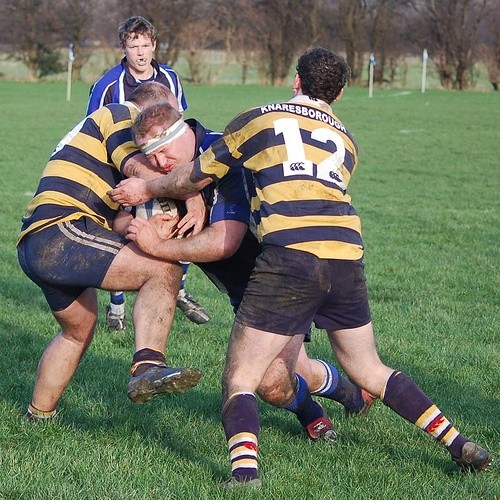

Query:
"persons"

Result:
[[113, 103, 377, 443], [17, 82, 207, 422], [87, 17, 211, 331], [106, 47, 492, 488]]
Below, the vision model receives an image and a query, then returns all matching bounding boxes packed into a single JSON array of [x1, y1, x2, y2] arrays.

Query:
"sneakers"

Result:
[[298, 401, 337, 442], [345, 388, 377, 419], [126, 366, 202, 405], [220, 476, 262, 489], [451, 442, 492, 476], [176, 290, 212, 325], [105, 305, 126, 333]]
